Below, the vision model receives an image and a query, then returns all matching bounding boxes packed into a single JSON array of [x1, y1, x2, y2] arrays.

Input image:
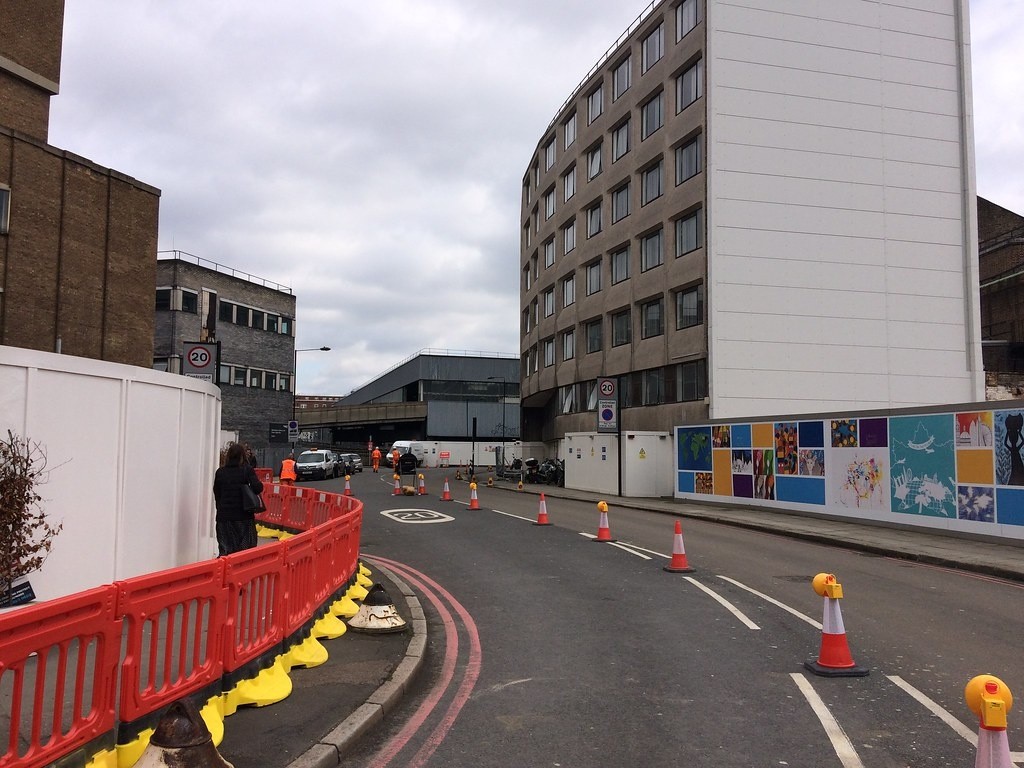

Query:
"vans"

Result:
[[295, 447, 335, 480], [386, 440, 424, 468]]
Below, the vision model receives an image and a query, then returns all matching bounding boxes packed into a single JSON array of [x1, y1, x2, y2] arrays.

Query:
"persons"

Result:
[[245, 449, 256, 468], [278, 452, 299, 486], [371, 446, 382, 473], [392, 446, 401, 473], [213, 444, 263, 595]]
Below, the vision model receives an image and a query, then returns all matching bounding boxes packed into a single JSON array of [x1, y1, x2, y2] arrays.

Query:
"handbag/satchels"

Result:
[[242, 483, 266, 513]]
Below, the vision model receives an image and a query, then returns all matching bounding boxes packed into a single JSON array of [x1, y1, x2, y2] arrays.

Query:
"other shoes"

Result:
[[373, 469, 375, 472], [376, 470, 378, 473]]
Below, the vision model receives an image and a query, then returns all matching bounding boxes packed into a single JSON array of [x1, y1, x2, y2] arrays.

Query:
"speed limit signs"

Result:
[[188, 347, 211, 368], [600, 380, 615, 396]]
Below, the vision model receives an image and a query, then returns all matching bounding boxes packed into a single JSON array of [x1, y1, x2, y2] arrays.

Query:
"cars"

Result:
[[347, 454, 363, 473], [331, 452, 347, 479], [340, 454, 356, 476]]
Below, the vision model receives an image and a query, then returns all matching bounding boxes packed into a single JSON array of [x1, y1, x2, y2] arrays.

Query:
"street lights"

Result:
[[291, 347, 333, 462], [486, 376, 506, 472]]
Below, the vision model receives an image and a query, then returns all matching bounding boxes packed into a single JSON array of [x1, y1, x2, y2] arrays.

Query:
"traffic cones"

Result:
[[416, 474, 428, 496], [344, 475, 354, 497], [392, 474, 405, 496], [662, 519, 698, 574], [804, 572, 870, 677], [964, 675, 1021, 768], [439, 478, 453, 501], [591, 501, 618, 543], [532, 492, 553, 526], [466, 483, 483, 510]]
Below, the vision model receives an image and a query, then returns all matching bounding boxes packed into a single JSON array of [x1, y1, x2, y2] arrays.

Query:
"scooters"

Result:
[[506, 453, 566, 487]]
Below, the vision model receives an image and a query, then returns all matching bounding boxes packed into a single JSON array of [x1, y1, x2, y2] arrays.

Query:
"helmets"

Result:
[[375, 446, 379, 449], [392, 447, 397, 449]]
